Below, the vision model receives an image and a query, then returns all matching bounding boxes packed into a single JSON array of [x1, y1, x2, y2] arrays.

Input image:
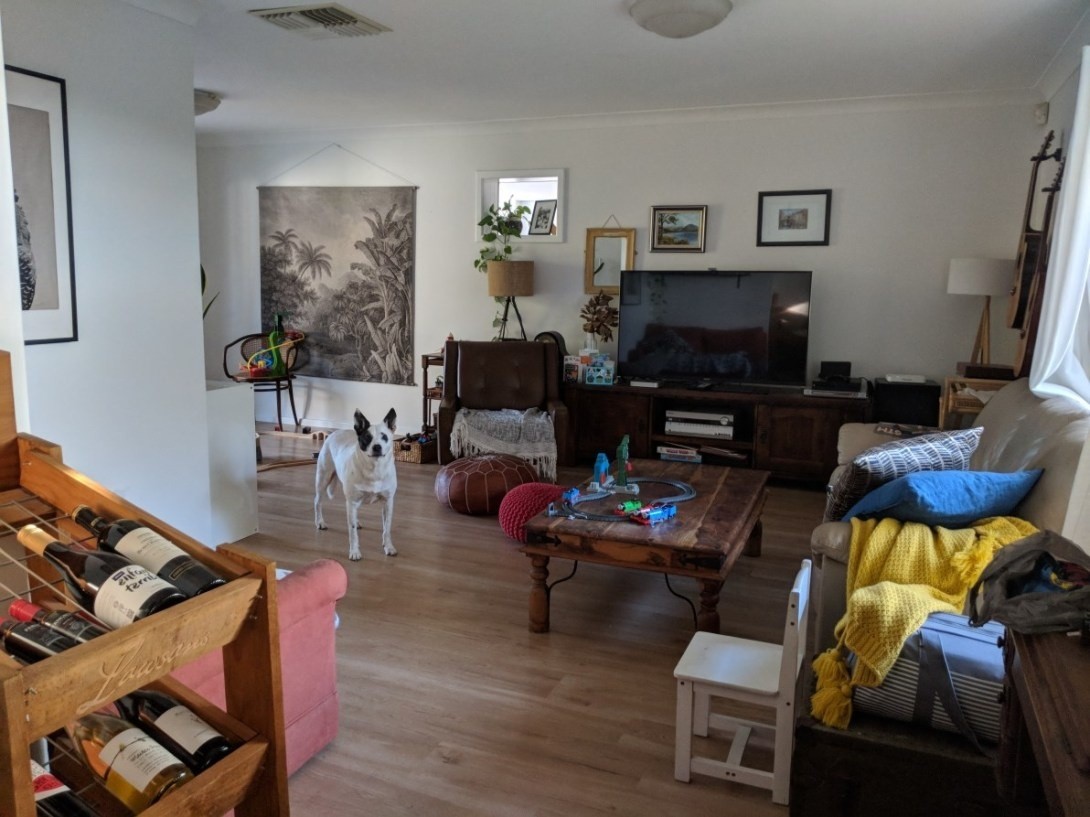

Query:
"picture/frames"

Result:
[[650, 205, 707, 253], [529, 200, 557, 235], [4, 63, 79, 345], [756, 189, 831, 246]]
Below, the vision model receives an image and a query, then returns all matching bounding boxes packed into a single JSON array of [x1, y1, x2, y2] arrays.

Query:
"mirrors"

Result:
[[586, 227, 637, 295]]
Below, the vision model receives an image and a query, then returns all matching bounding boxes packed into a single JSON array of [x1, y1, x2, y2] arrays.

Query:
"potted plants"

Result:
[[582, 290, 619, 350]]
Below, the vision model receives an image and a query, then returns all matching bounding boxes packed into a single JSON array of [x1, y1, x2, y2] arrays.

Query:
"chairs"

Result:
[[437, 341, 568, 485], [672, 560, 813, 805], [223, 332, 306, 432]]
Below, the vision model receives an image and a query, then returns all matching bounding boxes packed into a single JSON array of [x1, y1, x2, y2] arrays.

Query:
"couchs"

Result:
[[810, 378, 1090, 658], [168, 557, 347, 817]]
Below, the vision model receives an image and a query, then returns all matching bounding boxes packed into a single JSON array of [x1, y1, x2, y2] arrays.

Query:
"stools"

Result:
[[788, 663, 1001, 817]]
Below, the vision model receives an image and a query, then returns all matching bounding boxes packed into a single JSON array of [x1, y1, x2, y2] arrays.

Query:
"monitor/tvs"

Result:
[[618, 271, 812, 394]]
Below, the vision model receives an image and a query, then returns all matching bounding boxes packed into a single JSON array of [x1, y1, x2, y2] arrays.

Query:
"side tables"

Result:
[[939, 376, 1013, 430]]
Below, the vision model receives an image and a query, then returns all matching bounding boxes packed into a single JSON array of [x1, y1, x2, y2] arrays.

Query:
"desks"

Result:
[[875, 377, 941, 428], [997, 625, 1090, 817]]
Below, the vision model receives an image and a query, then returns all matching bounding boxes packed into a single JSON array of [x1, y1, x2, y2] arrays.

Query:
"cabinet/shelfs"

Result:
[[0, 349, 293, 817], [560, 383, 871, 493], [422, 355, 445, 433]]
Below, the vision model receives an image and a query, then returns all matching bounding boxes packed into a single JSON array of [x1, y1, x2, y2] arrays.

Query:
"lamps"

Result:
[[486, 261, 534, 341], [624, 0, 733, 40], [949, 258, 1020, 429], [193, 90, 221, 116]]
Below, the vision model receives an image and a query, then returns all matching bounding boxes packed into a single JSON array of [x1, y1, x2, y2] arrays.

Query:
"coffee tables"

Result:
[[518, 458, 772, 634]]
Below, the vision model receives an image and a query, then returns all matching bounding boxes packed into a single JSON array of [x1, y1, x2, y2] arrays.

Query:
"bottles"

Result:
[[114, 690, 238, 777], [17, 524, 188, 630], [64, 712, 194, 814], [8, 600, 108, 643], [71, 504, 227, 598], [0, 616, 80, 665], [30, 759, 103, 817]]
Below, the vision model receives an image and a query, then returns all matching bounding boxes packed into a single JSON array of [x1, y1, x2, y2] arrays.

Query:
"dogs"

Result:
[[314, 408, 398, 561]]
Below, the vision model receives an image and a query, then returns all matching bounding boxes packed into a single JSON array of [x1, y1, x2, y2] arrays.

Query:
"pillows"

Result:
[[823, 426, 1045, 528]]
